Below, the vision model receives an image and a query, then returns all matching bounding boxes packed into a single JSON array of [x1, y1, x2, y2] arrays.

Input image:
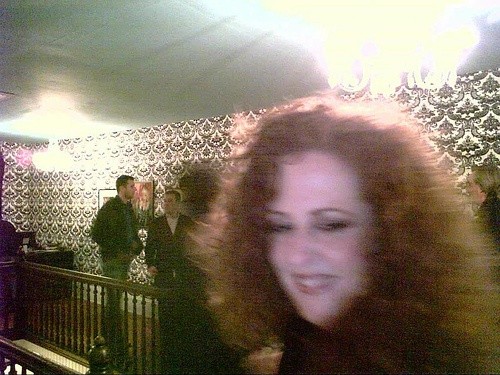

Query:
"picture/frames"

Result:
[[98, 188, 118, 211], [130, 180, 154, 227]]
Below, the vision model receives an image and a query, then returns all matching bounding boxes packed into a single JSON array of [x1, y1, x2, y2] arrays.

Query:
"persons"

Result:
[[186, 97, 498, 375], [90, 175, 143, 349], [174, 168, 245, 375], [147, 190, 192, 352], [470, 164, 500, 244]]
